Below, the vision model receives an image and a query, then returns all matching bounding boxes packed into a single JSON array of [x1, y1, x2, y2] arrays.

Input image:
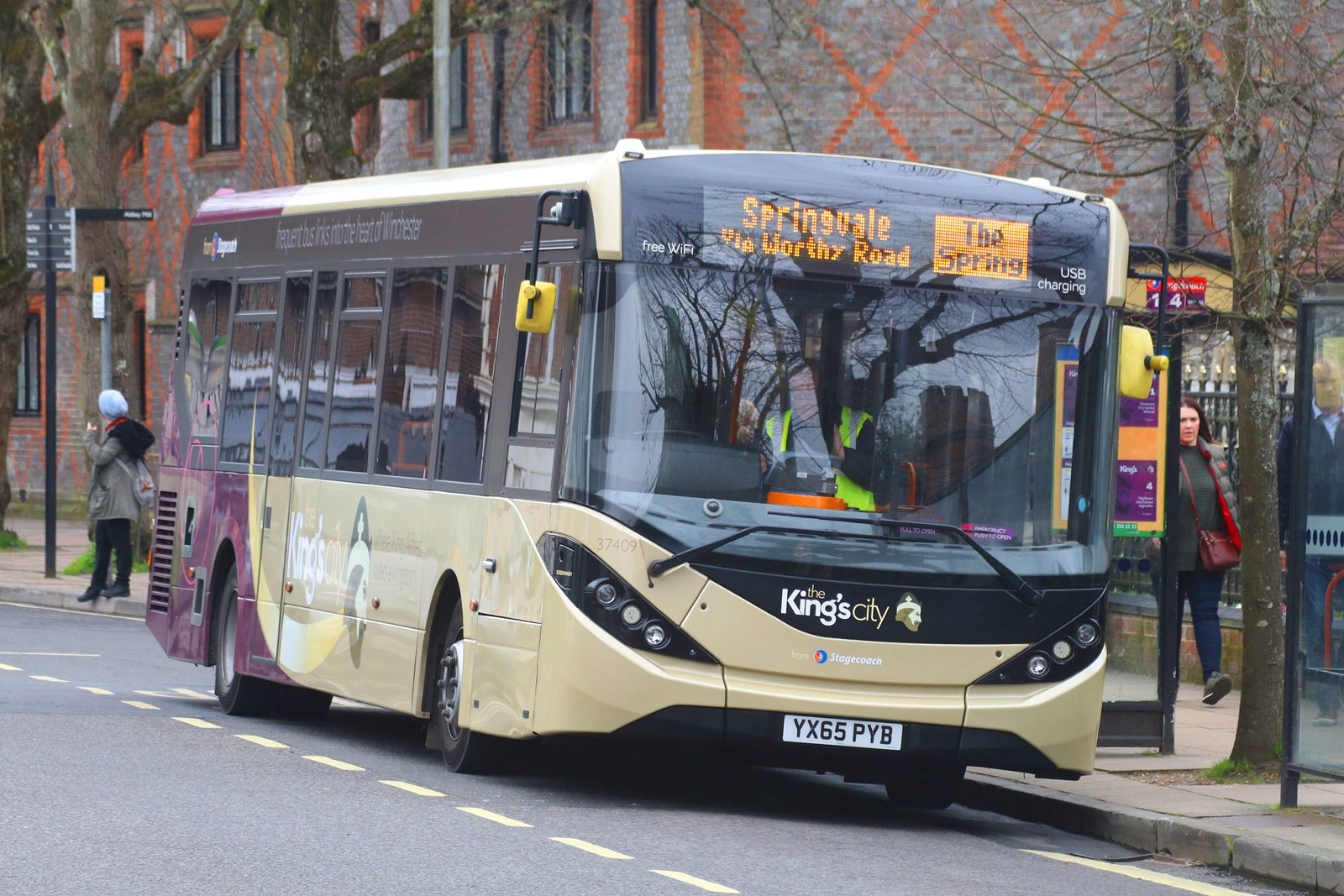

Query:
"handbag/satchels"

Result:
[[1198, 530, 1240, 571]]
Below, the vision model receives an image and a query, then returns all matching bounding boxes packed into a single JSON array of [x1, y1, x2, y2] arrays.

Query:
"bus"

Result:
[[143, 136, 1170, 809]]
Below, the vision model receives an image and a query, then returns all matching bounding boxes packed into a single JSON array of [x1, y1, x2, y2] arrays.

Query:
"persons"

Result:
[[1276, 359, 1344, 727], [76, 391, 155, 603], [1144, 395, 1241, 706], [746, 363, 895, 513]]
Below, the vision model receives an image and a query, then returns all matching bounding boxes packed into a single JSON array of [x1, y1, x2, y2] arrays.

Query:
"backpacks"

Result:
[[114, 452, 155, 507]]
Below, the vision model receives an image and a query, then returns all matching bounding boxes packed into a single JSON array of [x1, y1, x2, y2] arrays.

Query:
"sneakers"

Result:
[[1202, 671, 1232, 705]]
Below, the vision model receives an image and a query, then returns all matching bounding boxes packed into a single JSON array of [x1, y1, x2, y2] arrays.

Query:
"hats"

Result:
[[98, 390, 128, 420]]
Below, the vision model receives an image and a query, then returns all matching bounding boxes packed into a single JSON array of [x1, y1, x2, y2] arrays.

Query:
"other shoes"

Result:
[[78, 584, 108, 602], [99, 583, 129, 597], [1313, 707, 1338, 726]]
